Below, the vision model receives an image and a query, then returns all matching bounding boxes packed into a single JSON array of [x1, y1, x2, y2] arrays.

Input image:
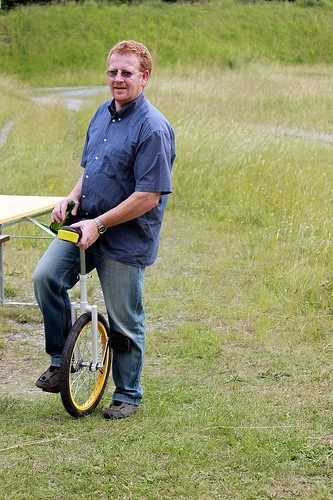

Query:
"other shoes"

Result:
[[103, 400, 139, 419], [35, 365, 62, 388]]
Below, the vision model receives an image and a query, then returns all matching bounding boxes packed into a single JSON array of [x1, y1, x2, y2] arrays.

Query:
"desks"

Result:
[[0, 194, 68, 307]]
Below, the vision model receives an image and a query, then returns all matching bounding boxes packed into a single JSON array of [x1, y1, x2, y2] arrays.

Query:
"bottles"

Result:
[[49, 200, 76, 234]]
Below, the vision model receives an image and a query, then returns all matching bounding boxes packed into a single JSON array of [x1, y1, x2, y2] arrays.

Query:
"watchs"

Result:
[[94, 218, 107, 234]]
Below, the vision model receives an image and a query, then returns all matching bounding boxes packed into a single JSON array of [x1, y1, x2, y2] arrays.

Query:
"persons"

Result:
[[32, 40, 177, 419]]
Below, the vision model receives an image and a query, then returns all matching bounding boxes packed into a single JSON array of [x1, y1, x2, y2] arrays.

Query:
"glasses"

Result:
[[106, 70, 143, 78]]
